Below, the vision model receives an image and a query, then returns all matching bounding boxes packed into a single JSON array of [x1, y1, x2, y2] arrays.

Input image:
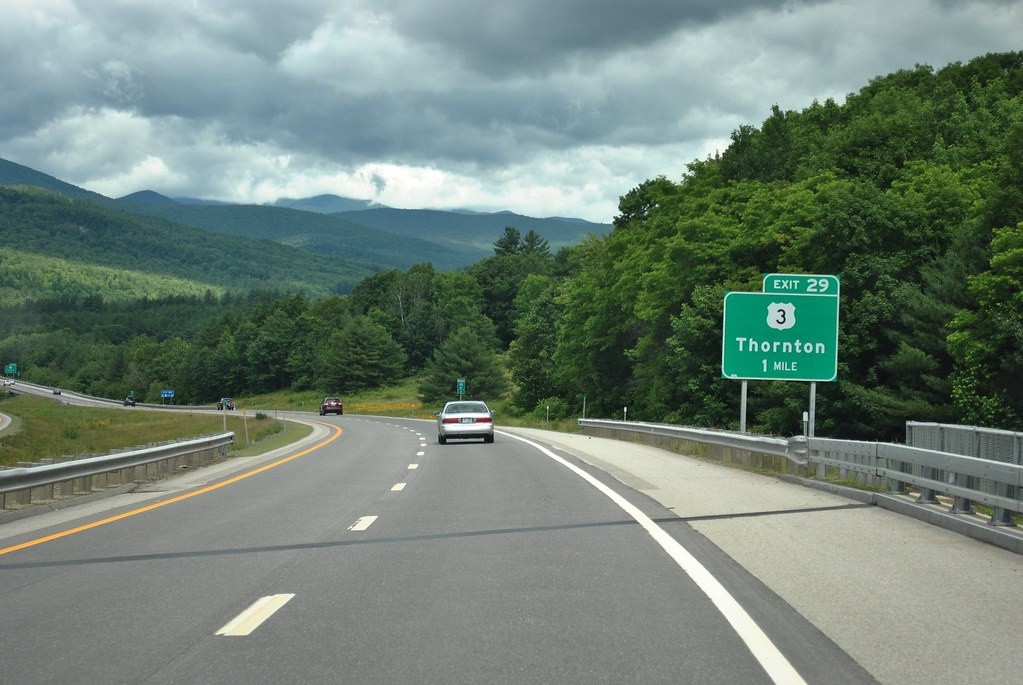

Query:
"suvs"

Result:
[[319, 397, 343, 416], [217, 398, 235, 411], [123, 397, 136, 407]]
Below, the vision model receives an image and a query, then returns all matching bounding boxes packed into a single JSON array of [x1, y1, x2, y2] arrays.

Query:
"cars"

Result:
[[433, 401, 496, 445], [53, 388, 61, 395], [3, 380, 15, 386]]
